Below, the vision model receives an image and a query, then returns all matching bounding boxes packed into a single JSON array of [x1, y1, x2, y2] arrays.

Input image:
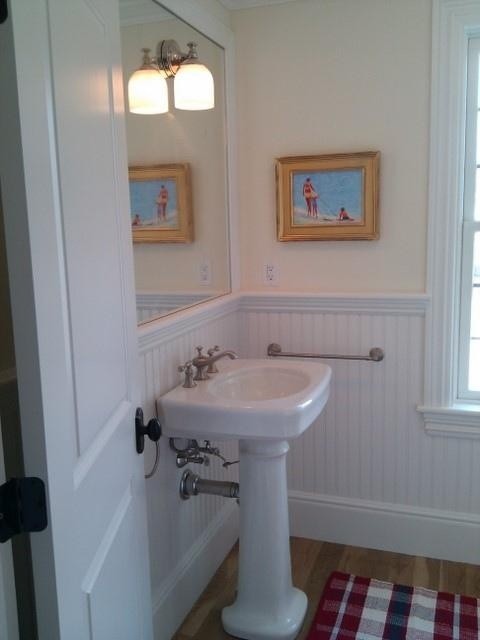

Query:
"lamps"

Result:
[[126, 46, 171, 117], [162, 39, 217, 115]]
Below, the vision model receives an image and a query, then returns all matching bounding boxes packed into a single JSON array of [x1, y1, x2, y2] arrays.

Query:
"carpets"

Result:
[[305, 566, 480, 640]]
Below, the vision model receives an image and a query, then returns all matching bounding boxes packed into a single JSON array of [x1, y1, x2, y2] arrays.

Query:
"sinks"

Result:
[[156, 359, 331, 440]]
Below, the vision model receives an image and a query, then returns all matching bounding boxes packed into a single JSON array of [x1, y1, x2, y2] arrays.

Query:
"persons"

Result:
[[336, 207, 354, 221], [132, 214, 140, 225], [156, 185, 168, 222], [302, 177, 319, 219]]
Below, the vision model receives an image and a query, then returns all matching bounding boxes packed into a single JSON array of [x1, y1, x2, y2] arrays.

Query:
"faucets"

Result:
[[193, 345, 239, 381]]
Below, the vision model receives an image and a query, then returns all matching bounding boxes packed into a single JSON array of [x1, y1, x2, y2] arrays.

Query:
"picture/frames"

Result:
[[275, 150, 383, 245], [128, 161, 194, 246]]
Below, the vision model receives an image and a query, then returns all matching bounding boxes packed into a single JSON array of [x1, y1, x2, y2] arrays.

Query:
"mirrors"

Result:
[[118, 1, 234, 329]]
